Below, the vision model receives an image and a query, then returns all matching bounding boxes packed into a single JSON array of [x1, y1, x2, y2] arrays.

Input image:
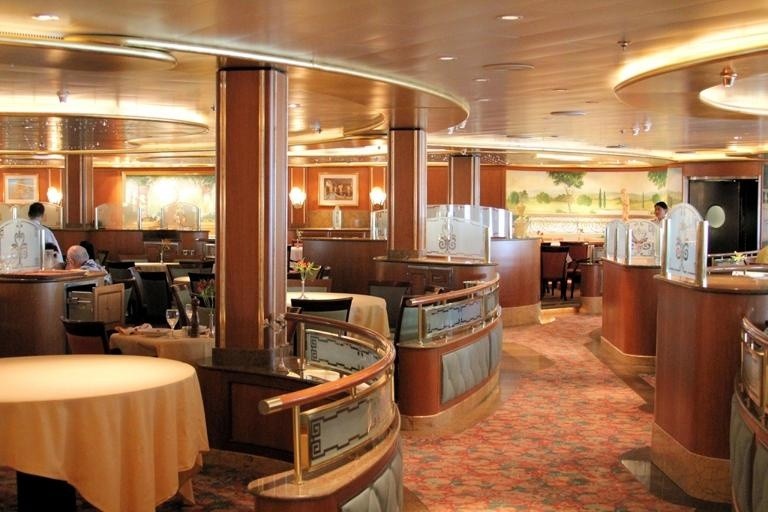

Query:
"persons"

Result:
[[649, 203, 667, 226], [323, 179, 353, 199], [61, 244, 113, 290], [28, 201, 64, 266], [618, 186, 631, 222]]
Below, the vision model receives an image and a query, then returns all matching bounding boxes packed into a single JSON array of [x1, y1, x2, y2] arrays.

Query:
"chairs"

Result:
[[288, 277, 447, 338], [539, 239, 586, 302], [80, 235, 217, 331]]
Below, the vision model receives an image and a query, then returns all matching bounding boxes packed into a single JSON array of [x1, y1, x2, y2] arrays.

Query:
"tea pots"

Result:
[[44, 249, 61, 271]]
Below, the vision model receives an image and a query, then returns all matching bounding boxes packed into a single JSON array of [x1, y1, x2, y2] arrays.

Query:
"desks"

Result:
[[0, 354, 213, 511]]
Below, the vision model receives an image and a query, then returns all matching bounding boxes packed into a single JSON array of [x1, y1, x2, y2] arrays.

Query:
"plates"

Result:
[[137, 328, 170, 337]]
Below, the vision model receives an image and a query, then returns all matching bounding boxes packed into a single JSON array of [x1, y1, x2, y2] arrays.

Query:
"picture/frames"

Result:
[[316, 170, 360, 207], [4, 171, 39, 204]]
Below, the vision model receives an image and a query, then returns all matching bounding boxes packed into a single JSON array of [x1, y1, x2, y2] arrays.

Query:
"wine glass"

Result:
[[164, 309, 181, 339], [185, 303, 193, 322]]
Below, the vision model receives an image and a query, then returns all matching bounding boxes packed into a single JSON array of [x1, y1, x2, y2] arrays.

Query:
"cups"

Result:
[[0, 250, 18, 274]]
